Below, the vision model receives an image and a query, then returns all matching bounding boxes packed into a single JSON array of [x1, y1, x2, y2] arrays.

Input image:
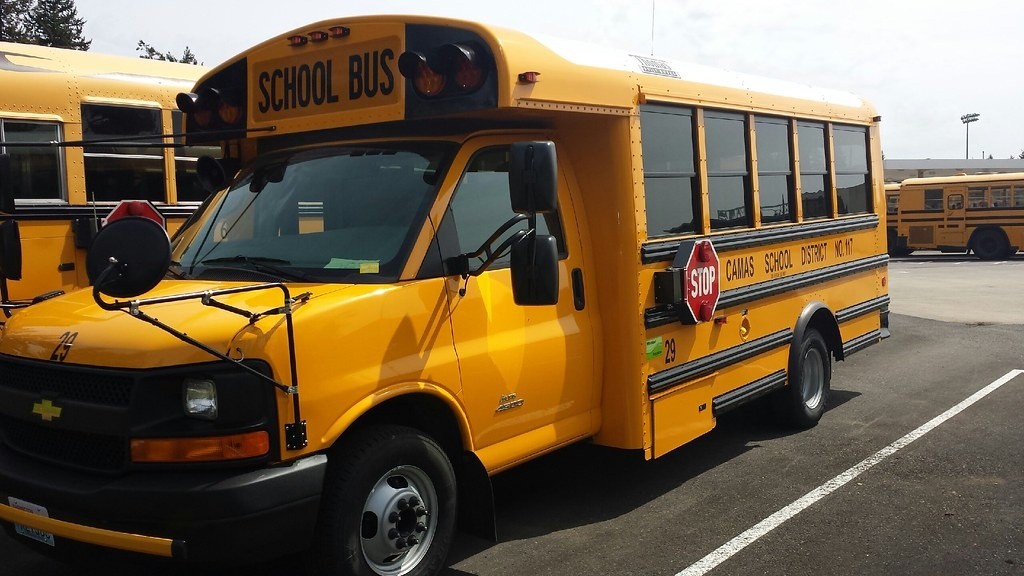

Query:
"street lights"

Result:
[[961, 112, 980, 159]]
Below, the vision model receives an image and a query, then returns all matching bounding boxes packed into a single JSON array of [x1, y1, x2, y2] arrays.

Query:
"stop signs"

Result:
[[685, 239, 722, 325]]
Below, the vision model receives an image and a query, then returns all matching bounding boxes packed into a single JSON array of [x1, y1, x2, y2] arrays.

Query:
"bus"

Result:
[[882, 183, 914, 256], [898, 171, 1024, 259], [0, 40, 331, 337], [0, 14, 892, 575]]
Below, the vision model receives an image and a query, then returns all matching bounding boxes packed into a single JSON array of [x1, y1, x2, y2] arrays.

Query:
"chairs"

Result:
[[325, 185, 381, 229]]
